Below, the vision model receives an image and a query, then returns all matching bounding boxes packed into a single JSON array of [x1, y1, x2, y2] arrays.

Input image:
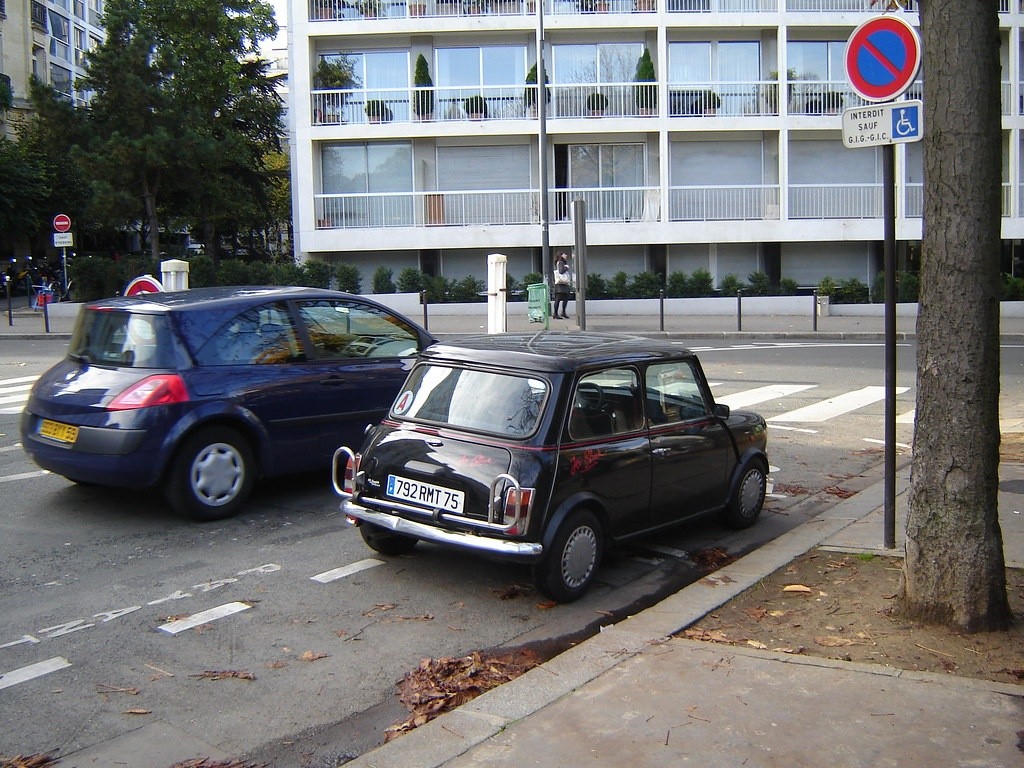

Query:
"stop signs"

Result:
[[53, 214, 71, 232]]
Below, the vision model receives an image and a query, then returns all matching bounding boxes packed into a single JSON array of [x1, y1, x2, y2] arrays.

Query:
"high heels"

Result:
[[553, 314, 563, 319], [563, 313, 569, 318]]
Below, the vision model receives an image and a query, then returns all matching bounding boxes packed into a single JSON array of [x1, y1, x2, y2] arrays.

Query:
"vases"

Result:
[[468, 0, 483, 14], [635, 0, 654, 11], [409, 3, 426, 16], [597, 0, 609, 11]]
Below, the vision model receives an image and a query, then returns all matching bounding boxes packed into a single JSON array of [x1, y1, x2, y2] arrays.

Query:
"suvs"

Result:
[[21, 284, 441, 520]]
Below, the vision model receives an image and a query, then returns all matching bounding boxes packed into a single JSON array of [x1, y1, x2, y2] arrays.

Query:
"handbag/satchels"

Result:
[[553, 262, 571, 286]]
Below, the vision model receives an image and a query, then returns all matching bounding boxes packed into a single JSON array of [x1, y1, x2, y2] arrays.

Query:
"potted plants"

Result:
[[316, 51, 365, 124], [698, 91, 721, 117], [587, 93, 608, 117], [635, 48, 657, 116], [464, 96, 487, 121], [762, 69, 797, 116], [366, 100, 386, 124], [413, 54, 434, 123], [806, 92, 843, 116], [524, 57, 551, 120], [315, 0, 345, 20], [355, 0, 382, 17]]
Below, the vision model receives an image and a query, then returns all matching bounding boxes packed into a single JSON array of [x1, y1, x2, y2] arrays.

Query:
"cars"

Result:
[[332, 329, 769, 604]]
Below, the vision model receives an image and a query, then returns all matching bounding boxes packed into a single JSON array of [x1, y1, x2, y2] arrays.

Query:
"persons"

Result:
[[238, 312, 260, 340], [552, 250, 571, 319]]
